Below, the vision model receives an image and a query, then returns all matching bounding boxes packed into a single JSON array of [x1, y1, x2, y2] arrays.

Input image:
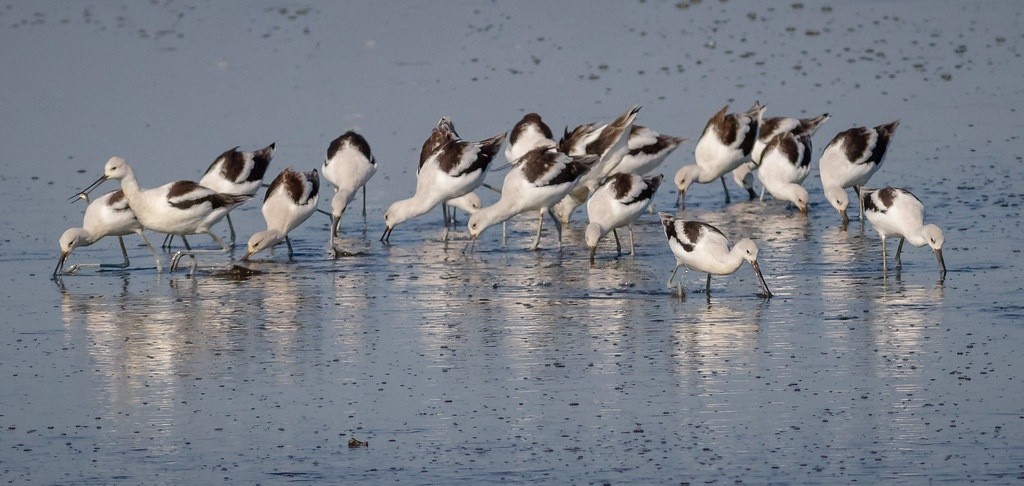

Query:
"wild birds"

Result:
[[818, 118, 899, 232], [51, 130, 378, 277], [658, 210, 773, 299], [673, 100, 832, 218], [380, 106, 687, 269], [860, 186, 947, 284]]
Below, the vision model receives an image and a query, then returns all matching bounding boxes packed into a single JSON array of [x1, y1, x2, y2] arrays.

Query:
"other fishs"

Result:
[[78, 192, 89, 202]]
